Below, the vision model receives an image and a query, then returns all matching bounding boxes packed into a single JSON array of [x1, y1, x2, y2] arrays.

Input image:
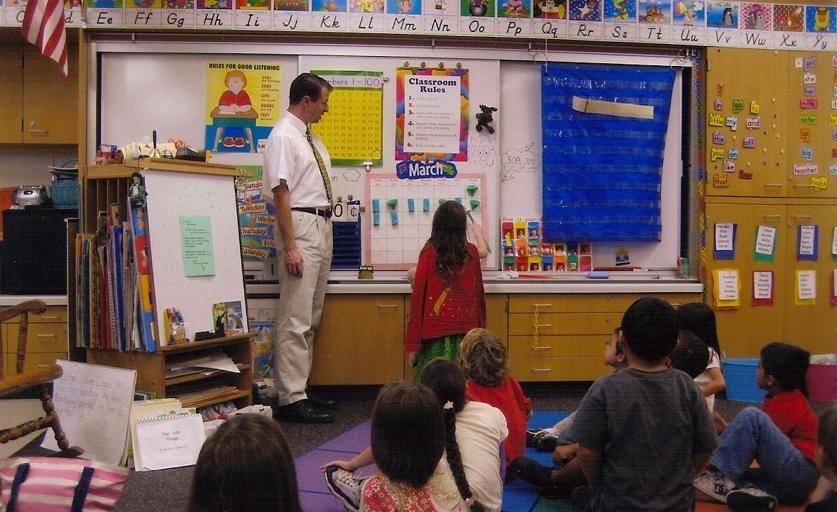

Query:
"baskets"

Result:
[[50, 158, 80, 208]]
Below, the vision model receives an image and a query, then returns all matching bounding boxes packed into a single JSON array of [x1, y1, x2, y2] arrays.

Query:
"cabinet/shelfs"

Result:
[[701, 47, 836, 198], [1, 42, 80, 144], [0, 305, 67, 385], [74, 156, 258, 421], [507, 293, 704, 385], [295, 294, 507, 388], [698, 204, 837, 358]]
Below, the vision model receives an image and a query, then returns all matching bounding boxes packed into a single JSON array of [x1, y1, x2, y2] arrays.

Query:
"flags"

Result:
[[23, 1, 70, 83]]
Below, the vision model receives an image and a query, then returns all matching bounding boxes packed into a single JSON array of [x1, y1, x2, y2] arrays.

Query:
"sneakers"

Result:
[[526, 428, 559, 451], [726, 484, 778, 512], [692, 470, 740, 504], [325, 465, 370, 511]]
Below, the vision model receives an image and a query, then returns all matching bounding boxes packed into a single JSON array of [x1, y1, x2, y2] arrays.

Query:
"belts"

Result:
[[291, 207, 333, 219]]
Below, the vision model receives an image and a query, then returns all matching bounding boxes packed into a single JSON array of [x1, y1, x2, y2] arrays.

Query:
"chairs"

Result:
[[0, 301, 83, 462]]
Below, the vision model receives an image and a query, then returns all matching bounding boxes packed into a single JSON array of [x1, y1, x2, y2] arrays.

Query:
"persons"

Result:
[[502, 228, 580, 272], [405, 201, 486, 382], [358, 375, 465, 512], [261, 71, 343, 423], [462, 327, 533, 476], [320, 357, 510, 512], [182, 415, 303, 511], [508, 297, 835, 512]]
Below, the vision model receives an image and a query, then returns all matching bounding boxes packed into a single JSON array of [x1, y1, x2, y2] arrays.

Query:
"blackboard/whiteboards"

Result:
[[100, 52, 682, 271]]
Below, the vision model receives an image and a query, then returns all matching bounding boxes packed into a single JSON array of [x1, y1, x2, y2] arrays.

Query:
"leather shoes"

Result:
[[303, 390, 337, 411], [277, 402, 336, 423]]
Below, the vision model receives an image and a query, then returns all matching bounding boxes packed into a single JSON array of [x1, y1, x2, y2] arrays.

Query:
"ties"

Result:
[[305, 126, 334, 206]]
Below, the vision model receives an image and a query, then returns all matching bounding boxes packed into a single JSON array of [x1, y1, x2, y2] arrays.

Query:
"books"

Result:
[[38, 195, 242, 468]]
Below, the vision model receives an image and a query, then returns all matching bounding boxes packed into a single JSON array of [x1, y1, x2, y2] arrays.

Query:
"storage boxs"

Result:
[[723, 359, 772, 406], [803, 364, 837, 405]]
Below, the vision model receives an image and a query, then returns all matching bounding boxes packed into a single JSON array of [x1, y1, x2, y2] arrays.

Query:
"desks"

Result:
[[209, 107, 257, 153]]
